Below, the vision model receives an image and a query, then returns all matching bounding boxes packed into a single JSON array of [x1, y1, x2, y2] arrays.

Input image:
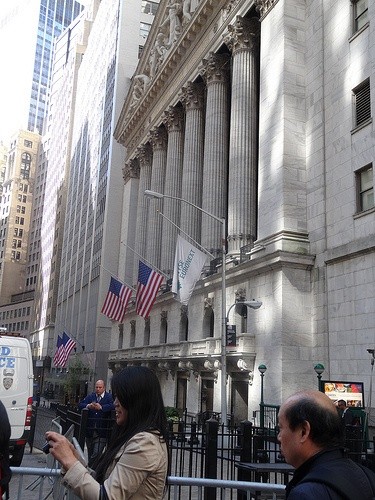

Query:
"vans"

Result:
[[0, 327, 35, 468]]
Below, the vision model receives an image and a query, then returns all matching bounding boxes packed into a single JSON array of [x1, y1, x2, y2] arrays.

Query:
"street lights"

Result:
[[314, 363, 326, 391], [143, 188, 228, 434], [257, 363, 267, 428], [221, 300, 264, 434]]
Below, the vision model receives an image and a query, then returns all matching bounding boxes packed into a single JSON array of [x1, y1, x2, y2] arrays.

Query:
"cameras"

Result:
[[41, 438, 55, 454]]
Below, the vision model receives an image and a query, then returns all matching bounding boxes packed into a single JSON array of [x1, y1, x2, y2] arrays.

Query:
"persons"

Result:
[[0, 402, 11, 500], [337, 400, 352, 454], [78, 380, 116, 471], [45, 367, 169, 500], [277, 389, 375, 500]]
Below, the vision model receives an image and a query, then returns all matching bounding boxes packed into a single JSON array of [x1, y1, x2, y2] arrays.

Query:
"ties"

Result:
[[96, 395, 102, 402]]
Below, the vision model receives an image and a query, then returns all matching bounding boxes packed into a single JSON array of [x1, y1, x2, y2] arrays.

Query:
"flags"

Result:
[[171, 234, 208, 306], [53, 331, 77, 368], [135, 260, 165, 320], [100, 276, 133, 323]]
